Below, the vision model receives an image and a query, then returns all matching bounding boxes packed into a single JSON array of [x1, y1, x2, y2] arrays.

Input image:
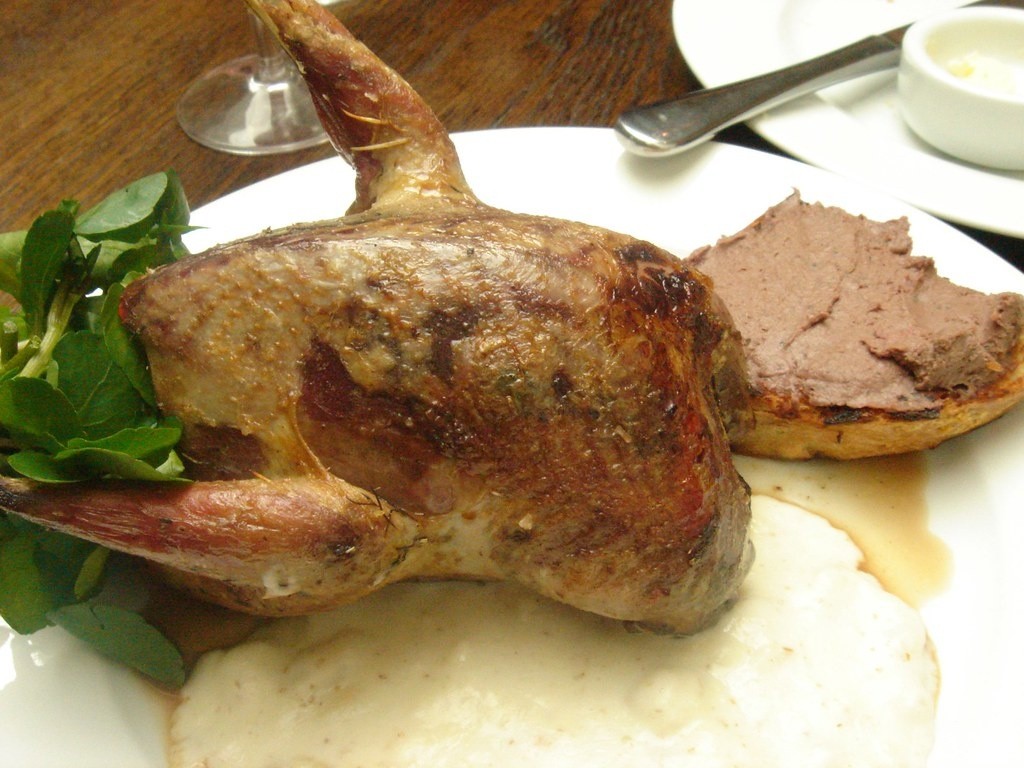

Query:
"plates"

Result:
[[671, 1, 1024, 241], [3, 125, 1024, 765]]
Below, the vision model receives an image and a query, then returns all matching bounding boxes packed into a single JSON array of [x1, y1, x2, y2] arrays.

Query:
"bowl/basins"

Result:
[[898, 5, 1024, 173]]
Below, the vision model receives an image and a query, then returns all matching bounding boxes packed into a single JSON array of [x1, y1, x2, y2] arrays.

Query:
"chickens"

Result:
[[0, 0, 758, 638]]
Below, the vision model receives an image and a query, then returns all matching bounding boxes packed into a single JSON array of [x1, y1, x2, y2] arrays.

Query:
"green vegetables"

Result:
[[0, 167, 203, 686]]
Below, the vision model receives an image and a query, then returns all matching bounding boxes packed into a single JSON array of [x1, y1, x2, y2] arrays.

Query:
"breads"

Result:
[[671, 191, 1024, 462]]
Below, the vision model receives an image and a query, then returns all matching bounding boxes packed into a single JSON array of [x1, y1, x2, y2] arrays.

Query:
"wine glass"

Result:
[[175, 0, 332, 156]]
